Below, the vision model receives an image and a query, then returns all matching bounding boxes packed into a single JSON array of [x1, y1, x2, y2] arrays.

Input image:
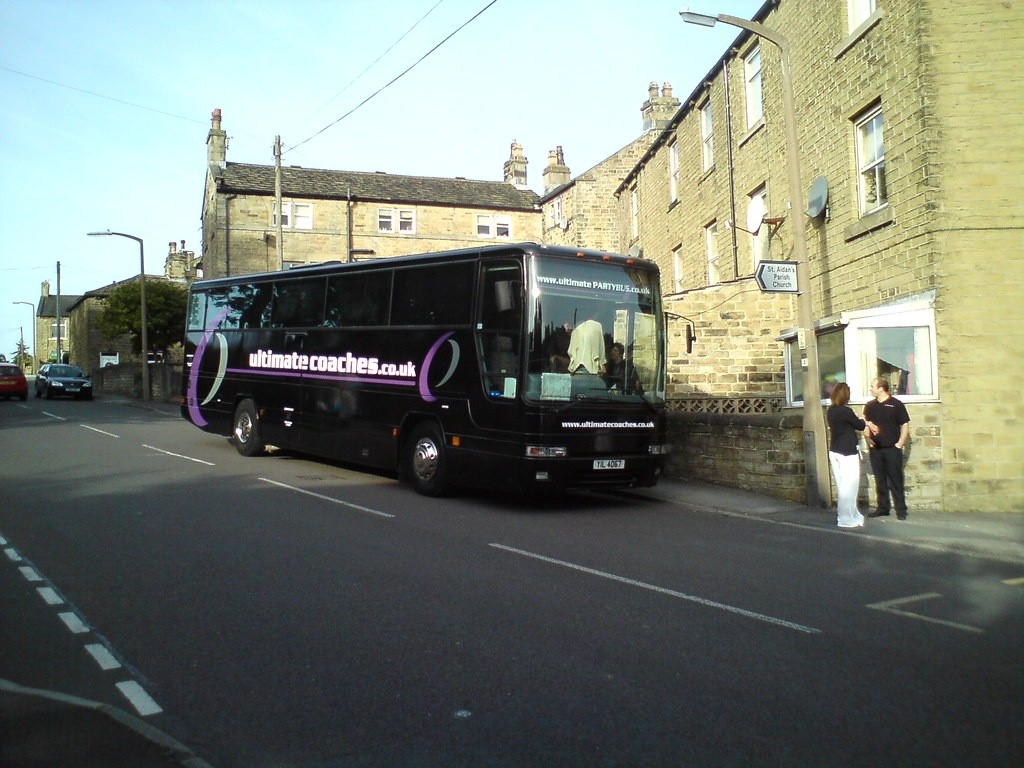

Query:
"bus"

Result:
[[0, 353, 6, 363], [179, 242, 697, 501]]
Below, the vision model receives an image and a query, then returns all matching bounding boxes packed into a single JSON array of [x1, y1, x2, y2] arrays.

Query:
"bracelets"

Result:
[[894, 443, 902, 448], [866, 421, 869, 426]]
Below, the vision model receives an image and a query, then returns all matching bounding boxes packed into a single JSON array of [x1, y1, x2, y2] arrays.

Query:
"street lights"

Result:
[[678, 8, 833, 511], [85, 229, 151, 404], [12, 300, 36, 375]]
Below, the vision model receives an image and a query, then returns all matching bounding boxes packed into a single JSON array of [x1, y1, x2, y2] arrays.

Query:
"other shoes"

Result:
[[896, 507, 906, 520], [868, 510, 889, 517]]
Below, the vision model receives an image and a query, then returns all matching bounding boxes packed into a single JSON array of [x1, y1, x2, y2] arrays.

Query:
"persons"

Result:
[[531, 306, 641, 388], [829, 382, 879, 528], [862, 378, 911, 520]]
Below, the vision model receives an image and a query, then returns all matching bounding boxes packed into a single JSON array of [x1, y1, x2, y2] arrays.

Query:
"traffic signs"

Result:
[[755, 260, 797, 294]]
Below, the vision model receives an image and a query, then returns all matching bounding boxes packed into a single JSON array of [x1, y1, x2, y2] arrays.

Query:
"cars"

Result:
[[34, 362, 93, 402], [0, 363, 29, 402]]
